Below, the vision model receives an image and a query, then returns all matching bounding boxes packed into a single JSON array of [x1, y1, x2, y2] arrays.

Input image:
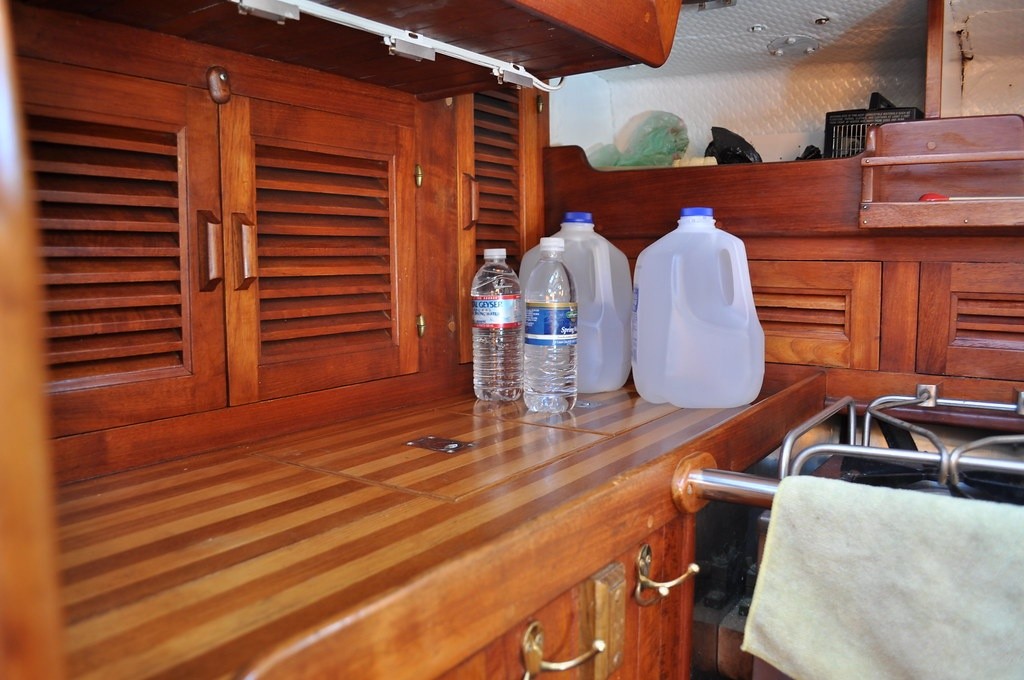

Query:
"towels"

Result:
[[740, 474, 1023, 680]]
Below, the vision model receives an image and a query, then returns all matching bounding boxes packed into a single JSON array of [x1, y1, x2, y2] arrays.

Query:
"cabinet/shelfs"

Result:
[[4, 52, 425, 442], [436, 514, 692, 680]]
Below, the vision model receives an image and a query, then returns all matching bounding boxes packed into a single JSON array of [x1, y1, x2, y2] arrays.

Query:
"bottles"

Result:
[[471, 248, 523, 401], [518, 210, 631, 395], [631, 207, 765, 409], [524, 237, 578, 413]]
